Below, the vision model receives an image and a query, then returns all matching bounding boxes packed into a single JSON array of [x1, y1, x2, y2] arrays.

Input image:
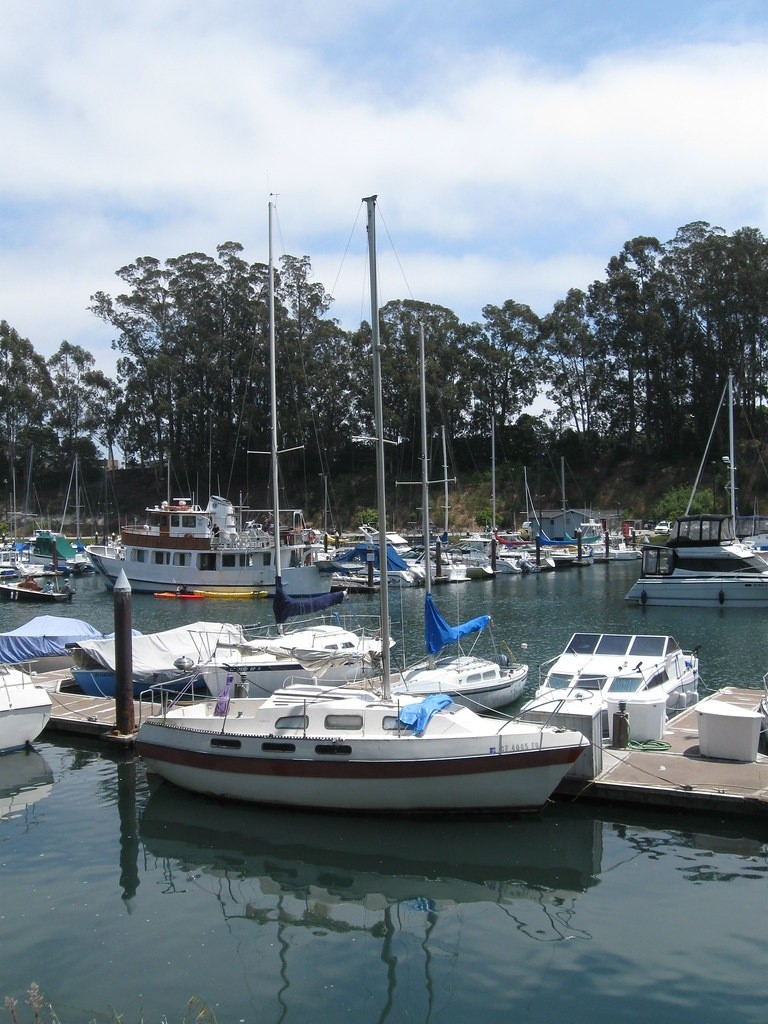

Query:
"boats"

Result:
[[1, 745, 55, 825], [138, 785, 605, 944], [0, 615, 701, 814]]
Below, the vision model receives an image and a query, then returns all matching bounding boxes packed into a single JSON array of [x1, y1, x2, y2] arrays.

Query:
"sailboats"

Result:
[[0, 196, 767, 607]]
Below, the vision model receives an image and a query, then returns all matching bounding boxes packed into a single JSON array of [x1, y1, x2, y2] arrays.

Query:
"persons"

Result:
[[262, 522, 272, 547], [210, 524, 220, 550], [43, 580, 54, 593], [60, 579, 71, 594]]
